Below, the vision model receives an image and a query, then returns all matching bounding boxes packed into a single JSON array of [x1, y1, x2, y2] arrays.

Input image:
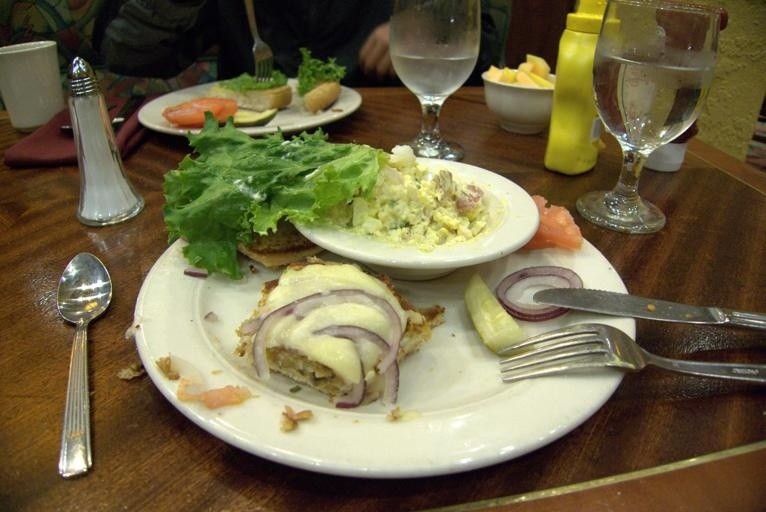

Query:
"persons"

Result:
[[90, 1, 514, 87]]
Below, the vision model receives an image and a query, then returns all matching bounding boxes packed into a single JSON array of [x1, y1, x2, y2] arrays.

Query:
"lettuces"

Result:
[[159, 107, 397, 282]]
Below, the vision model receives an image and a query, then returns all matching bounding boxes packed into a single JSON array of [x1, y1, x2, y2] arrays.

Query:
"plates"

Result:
[[296, 155, 537, 278], [137, 77, 362, 138], [134, 237, 636, 482]]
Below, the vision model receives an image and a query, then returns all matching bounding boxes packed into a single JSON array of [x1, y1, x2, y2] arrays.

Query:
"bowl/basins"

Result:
[[482, 70, 556, 137]]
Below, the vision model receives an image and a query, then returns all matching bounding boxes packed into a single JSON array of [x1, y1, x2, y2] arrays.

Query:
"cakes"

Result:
[[233, 257, 451, 411]]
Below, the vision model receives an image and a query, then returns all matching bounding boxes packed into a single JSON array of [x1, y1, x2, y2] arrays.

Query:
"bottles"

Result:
[[61, 56, 144, 231], [641, 6, 731, 174], [544, 2, 622, 177]]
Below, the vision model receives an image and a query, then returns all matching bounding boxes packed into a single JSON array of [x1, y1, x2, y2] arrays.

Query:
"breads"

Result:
[[233, 225, 322, 270], [302, 80, 342, 114], [206, 81, 294, 112]]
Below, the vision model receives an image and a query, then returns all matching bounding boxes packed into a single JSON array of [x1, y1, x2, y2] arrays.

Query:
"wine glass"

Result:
[[578, 1, 723, 234], [389, 0, 483, 160]]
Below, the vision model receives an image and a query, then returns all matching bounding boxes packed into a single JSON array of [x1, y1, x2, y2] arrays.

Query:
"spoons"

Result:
[[54, 251, 113, 476]]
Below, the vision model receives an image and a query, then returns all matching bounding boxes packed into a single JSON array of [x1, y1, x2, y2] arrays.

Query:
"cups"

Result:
[[1, 38, 64, 131]]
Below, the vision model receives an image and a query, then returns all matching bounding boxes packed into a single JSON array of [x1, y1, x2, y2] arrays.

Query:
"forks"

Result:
[[241, 1, 274, 82], [499, 321, 765, 396]]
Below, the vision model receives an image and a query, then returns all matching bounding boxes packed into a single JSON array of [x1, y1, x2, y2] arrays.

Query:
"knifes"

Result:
[[534, 286, 765, 340]]
